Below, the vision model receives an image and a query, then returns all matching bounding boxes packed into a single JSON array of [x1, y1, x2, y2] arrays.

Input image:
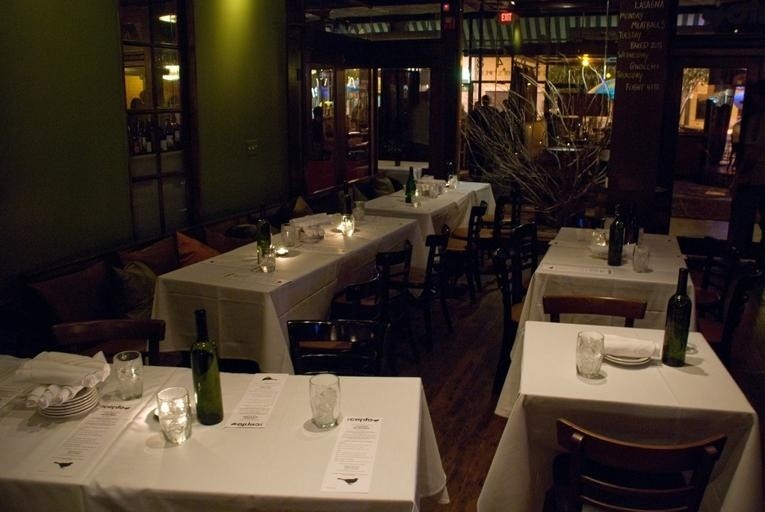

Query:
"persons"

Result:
[[727, 121, 741, 171], [126, 97, 141, 127], [309, 107, 328, 160], [467, 94, 499, 178]]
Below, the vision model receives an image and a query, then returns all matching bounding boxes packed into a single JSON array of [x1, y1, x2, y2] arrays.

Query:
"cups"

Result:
[[156, 385, 193, 442], [411, 196, 419, 208], [259, 246, 275, 271], [591, 228, 605, 258], [281, 223, 295, 246], [352, 207, 364, 232], [414, 190, 420, 196], [309, 373, 340, 428], [429, 184, 439, 198], [633, 245, 650, 272], [342, 214, 354, 235], [112, 349, 144, 400], [576, 332, 603, 378], [403, 184, 406, 191]]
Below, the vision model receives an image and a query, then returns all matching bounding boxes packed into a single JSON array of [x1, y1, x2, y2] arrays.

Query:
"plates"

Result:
[[38, 388, 97, 418], [604, 354, 651, 366]]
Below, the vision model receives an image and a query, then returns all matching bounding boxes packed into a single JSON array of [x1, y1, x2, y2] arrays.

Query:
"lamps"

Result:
[[582, 40, 590, 66]]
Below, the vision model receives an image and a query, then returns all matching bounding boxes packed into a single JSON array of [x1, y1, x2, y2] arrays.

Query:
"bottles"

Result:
[[448, 162, 454, 174], [341, 181, 353, 215], [256, 202, 271, 265], [624, 202, 638, 243], [405, 166, 416, 203], [190, 308, 227, 425], [608, 204, 625, 266], [662, 267, 692, 367]]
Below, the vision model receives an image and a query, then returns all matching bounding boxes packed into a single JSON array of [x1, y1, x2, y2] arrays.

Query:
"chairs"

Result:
[[727, 134, 738, 171]]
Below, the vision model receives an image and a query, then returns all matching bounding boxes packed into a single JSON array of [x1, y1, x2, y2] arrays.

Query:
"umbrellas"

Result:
[[709, 86, 745, 110], [586, 78, 616, 98]]
